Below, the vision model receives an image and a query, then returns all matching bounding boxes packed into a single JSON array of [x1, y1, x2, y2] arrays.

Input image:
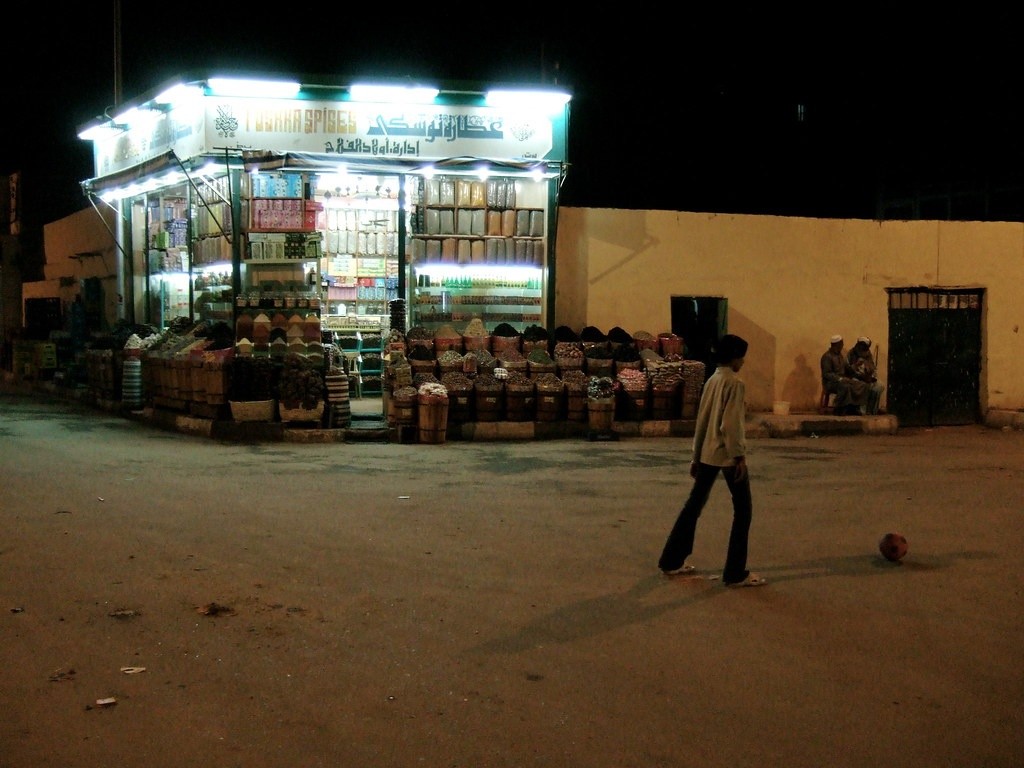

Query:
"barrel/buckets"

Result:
[[234, 294, 323, 365], [377, 333, 704, 444], [125, 348, 140, 359], [771, 400, 791, 415]]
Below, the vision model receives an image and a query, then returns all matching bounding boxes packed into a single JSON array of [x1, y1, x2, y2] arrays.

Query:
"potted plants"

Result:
[[279, 352, 325, 424], [225, 369, 278, 424]]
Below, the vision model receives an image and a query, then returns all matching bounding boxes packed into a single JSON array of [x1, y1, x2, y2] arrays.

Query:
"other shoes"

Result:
[[727, 571, 768, 586], [664, 564, 695, 574]]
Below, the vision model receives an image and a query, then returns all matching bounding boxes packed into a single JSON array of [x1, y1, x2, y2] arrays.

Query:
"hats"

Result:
[[858, 337, 870, 347], [831, 334, 842, 343]]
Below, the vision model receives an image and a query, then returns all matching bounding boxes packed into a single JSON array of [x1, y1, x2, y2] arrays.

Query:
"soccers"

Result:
[[879, 532, 908, 560]]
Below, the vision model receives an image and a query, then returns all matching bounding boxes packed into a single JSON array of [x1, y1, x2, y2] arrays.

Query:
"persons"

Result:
[[846, 334, 885, 416], [820, 335, 862, 416], [656, 335, 768, 589]]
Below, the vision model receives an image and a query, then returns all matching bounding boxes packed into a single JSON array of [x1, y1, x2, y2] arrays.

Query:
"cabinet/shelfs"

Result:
[[127, 160, 551, 324]]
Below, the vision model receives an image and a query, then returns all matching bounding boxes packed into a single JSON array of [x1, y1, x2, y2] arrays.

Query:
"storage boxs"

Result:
[[146, 347, 230, 407], [383, 322, 705, 444]]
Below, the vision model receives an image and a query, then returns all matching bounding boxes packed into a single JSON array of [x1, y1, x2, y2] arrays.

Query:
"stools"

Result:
[[818, 388, 838, 416]]
